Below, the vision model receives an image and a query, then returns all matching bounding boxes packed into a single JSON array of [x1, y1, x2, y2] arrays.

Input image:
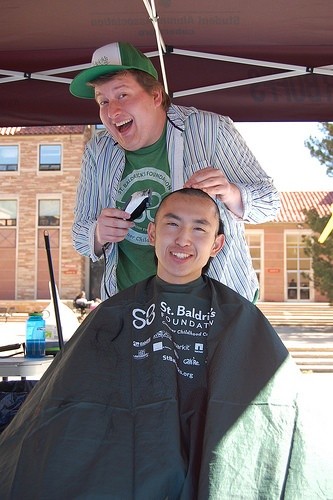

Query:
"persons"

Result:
[[68, 41, 280, 310], [1, 187, 333, 500]]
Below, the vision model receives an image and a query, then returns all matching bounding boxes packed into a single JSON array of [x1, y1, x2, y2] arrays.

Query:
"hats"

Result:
[[69, 42, 158, 100]]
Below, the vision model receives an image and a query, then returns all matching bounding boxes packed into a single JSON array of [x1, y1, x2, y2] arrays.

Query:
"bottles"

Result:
[[25, 310, 50, 358]]
